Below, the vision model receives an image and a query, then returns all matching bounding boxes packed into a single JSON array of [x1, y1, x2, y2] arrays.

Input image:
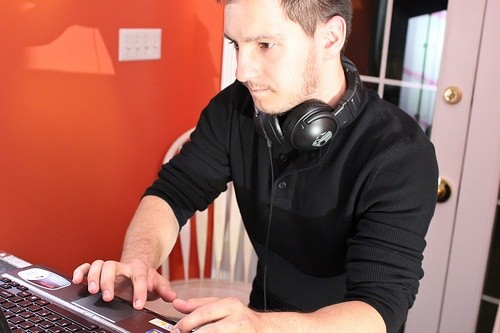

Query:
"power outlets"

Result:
[[118, 28, 163, 62]]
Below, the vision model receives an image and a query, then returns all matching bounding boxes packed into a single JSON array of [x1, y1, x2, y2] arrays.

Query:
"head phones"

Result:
[[253, 55, 364, 153]]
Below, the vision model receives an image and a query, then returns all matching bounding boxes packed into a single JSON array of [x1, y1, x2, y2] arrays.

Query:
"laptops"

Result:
[[0, 250, 196, 333]]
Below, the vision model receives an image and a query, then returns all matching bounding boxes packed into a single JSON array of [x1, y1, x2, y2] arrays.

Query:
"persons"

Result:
[[73, 0, 439, 333]]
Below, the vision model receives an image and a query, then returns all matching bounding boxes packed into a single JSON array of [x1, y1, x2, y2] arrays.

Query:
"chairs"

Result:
[[162, 127, 259, 307]]
